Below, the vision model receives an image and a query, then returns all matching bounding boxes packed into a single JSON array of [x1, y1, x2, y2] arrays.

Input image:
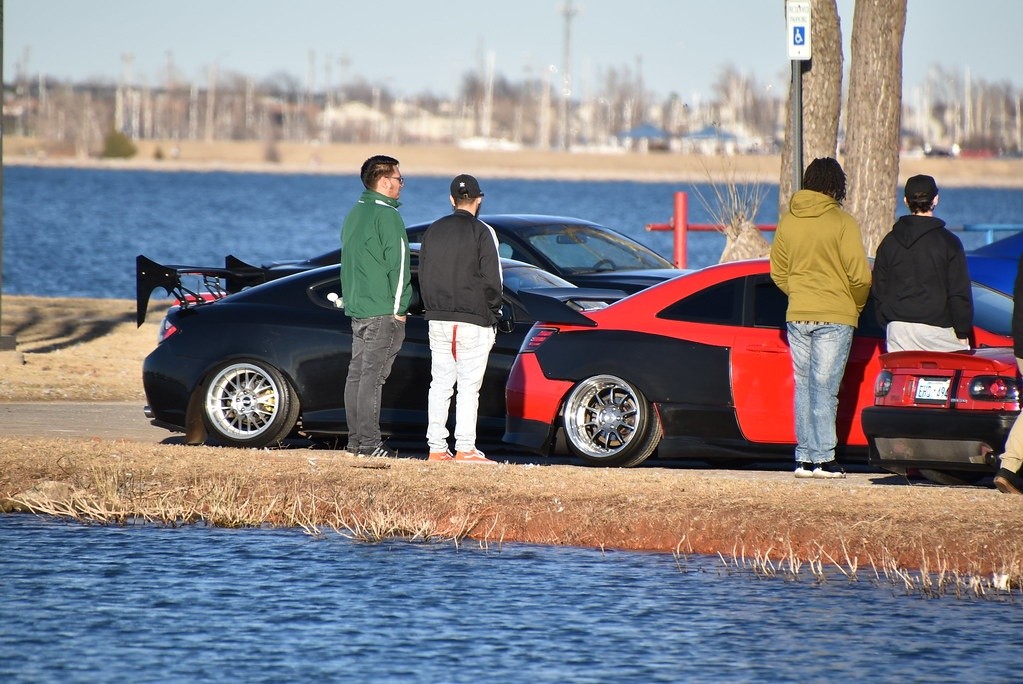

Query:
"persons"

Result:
[[419, 174, 503, 464], [769, 158, 872, 478], [994, 253, 1023, 493], [340, 157, 414, 457], [871, 174, 973, 352]]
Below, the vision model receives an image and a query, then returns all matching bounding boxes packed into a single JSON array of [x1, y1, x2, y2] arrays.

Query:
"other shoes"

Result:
[[994, 468, 1023, 494]]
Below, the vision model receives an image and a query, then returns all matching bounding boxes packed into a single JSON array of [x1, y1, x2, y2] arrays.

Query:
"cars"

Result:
[[263, 213, 696, 298], [502, 259, 1022, 466], [128, 254, 610, 452]]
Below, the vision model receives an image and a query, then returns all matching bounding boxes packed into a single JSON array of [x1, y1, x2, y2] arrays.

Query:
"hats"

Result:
[[451, 174, 484, 198], [905, 175, 939, 202]]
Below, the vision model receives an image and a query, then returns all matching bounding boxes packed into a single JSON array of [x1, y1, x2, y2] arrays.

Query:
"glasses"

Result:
[[383, 176, 404, 184]]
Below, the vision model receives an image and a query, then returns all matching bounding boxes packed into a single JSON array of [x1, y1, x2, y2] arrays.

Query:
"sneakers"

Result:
[[455, 449, 498, 465], [357, 444, 398, 459], [346, 448, 360, 458], [429, 450, 455, 463], [795, 461, 814, 478], [811, 460, 846, 478]]
